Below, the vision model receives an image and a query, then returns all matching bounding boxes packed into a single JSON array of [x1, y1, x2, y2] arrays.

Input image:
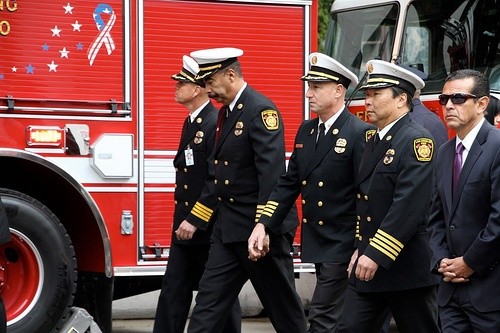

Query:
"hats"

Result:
[[171, 55, 206, 87], [190, 47, 244, 81], [358, 60, 426, 97], [300, 52, 358, 89]]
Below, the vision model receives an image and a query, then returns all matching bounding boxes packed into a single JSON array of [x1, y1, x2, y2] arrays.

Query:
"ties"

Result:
[[451, 142, 465, 192], [175, 116, 191, 159], [213, 105, 228, 148], [372, 133, 380, 150], [315, 124, 326, 150]]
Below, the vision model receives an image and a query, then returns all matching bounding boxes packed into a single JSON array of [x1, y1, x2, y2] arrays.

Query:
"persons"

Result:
[[187, 47, 308, 333], [152, 55, 243, 333], [247, 53, 380, 333], [332, 57, 499, 333]]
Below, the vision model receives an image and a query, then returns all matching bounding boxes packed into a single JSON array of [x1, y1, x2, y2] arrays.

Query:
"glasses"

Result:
[[438, 93, 477, 105]]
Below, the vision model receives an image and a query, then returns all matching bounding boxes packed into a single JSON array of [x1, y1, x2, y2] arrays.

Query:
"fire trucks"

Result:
[[311, 0, 500, 142], [0, 0, 318, 333]]
